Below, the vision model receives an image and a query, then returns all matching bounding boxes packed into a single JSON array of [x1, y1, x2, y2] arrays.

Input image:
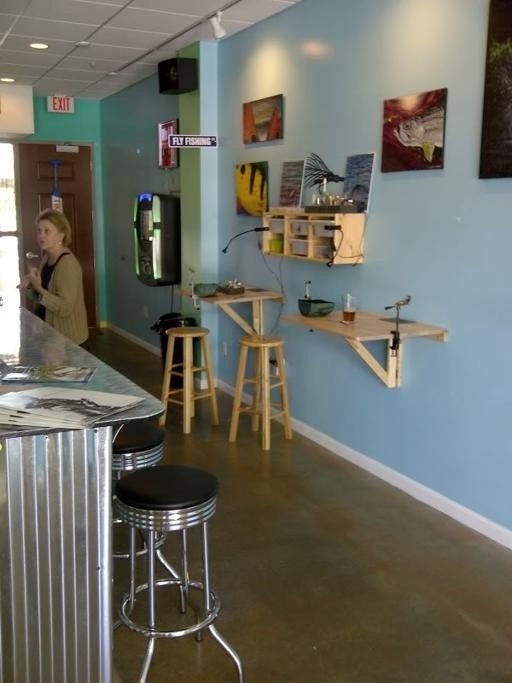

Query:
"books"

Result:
[[1, 365, 97, 383], [0, 387, 146, 430]]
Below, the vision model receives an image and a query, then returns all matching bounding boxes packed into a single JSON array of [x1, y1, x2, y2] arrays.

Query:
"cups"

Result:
[[269, 239, 282, 252], [341, 293, 358, 321]]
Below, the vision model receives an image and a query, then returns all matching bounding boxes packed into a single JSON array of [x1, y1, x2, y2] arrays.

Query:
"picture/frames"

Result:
[[157, 116, 179, 168]]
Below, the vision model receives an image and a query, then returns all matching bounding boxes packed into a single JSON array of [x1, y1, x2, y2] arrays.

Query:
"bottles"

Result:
[[311, 192, 354, 206]]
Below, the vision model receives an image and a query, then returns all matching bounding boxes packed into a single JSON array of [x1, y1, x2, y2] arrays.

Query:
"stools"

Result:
[[112, 465, 244, 683], [109, 422, 186, 614], [158, 326, 220, 434], [228, 333, 293, 451]]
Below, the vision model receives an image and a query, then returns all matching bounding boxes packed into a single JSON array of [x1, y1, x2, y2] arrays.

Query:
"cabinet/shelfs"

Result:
[[262, 211, 365, 266]]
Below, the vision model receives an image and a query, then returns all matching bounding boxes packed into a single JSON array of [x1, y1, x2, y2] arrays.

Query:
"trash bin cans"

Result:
[[149, 313, 199, 389]]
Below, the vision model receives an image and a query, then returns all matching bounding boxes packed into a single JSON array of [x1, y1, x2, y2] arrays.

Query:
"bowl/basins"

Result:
[[193, 283, 218, 296], [298, 298, 334, 316]]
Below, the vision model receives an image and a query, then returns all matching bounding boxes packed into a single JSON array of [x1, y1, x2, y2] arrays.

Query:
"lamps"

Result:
[[208, 15, 227, 40]]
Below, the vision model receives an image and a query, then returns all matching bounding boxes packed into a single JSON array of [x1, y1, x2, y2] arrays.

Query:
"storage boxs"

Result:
[[270, 239, 283, 253], [289, 218, 308, 236], [288, 238, 307, 255], [312, 219, 334, 238], [311, 246, 332, 259], [268, 218, 284, 234]]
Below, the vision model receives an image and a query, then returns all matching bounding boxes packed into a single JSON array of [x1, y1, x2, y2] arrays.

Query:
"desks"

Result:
[[178, 284, 285, 335], [281, 308, 448, 389]]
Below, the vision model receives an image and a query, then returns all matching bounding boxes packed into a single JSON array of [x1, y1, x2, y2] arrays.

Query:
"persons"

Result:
[[16, 208, 89, 346]]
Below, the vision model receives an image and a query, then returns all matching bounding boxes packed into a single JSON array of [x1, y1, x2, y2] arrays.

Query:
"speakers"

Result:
[[158, 57, 197, 94]]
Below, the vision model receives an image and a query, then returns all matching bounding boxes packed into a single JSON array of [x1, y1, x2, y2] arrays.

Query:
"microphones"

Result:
[[222, 227, 269, 253], [324, 224, 343, 267]]
